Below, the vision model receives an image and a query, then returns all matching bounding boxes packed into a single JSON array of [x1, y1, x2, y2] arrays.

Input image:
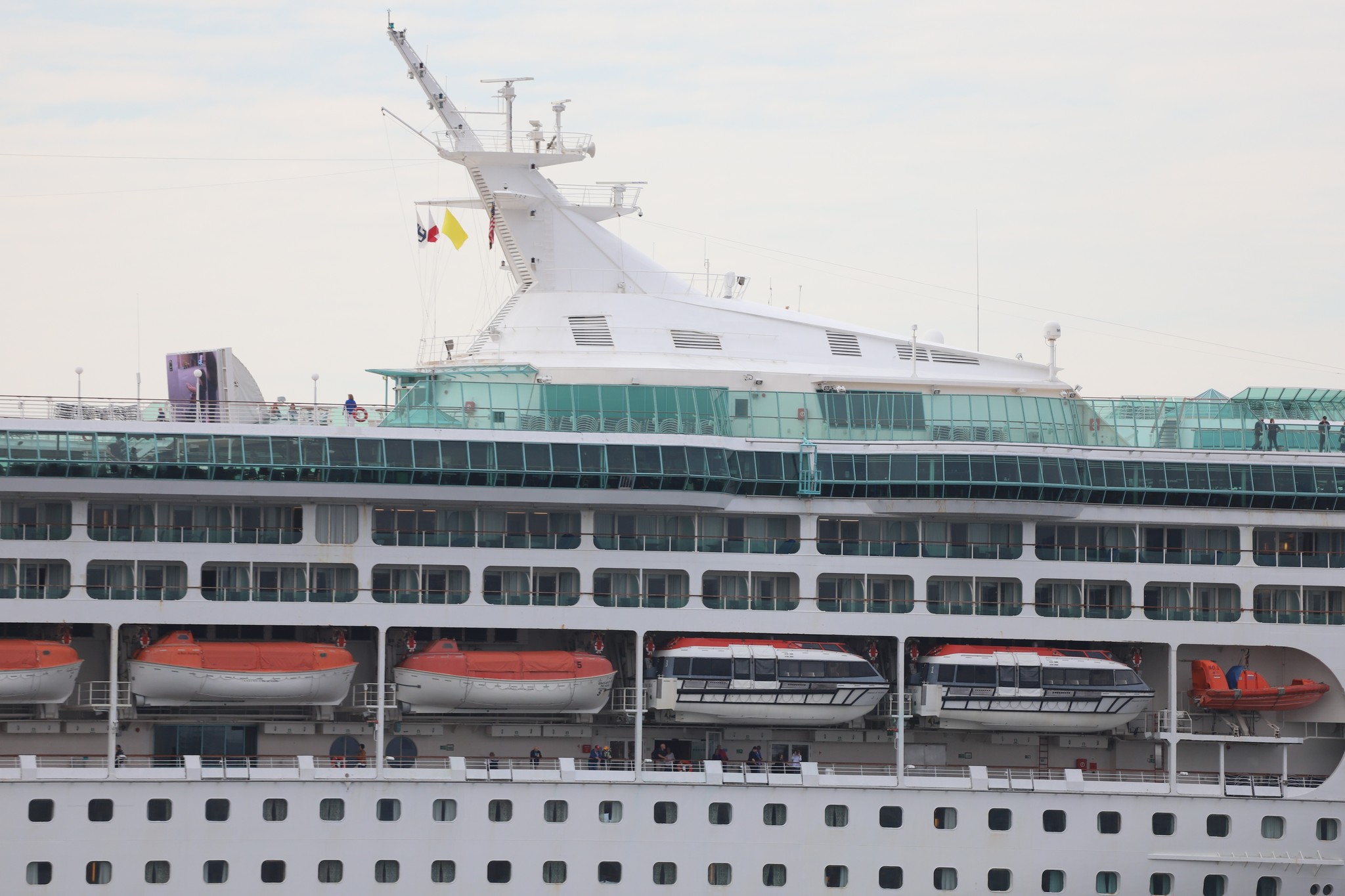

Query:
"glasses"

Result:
[[490, 754, 493, 756], [758, 749, 760, 751], [604, 749, 607, 750]]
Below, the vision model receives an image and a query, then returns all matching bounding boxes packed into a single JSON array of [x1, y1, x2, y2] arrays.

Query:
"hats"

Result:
[[595, 745, 600, 749], [1260, 418, 1264, 420], [603, 746, 608, 750], [716, 745, 721, 748], [1323, 416, 1327, 419]]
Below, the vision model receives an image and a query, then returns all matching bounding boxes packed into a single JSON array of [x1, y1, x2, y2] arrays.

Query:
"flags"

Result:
[[428, 209, 440, 242], [442, 208, 469, 250], [416, 209, 429, 247], [489, 201, 495, 249]]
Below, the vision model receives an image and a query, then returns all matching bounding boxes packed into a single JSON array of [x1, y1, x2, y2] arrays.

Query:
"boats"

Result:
[[389, 636, 617, 718], [0, 638, 84, 700], [126, 630, 360, 704], [644, 637, 891, 725], [913, 646, 1158, 733], [1183, 660, 1330, 712]]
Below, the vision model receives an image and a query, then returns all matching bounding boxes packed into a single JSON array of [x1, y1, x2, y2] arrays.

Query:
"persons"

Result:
[[1268, 418, 1281, 451], [187, 369, 206, 422], [342, 394, 357, 426], [156, 408, 165, 421], [1318, 416, 1331, 452], [1254, 417, 1268, 450], [269, 403, 298, 417], [107, 439, 137, 461], [115, 743, 802, 773], [1338, 422, 1345, 453]]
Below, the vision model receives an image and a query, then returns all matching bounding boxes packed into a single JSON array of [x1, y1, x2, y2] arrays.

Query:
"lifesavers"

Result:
[[331, 756, 346, 769], [352, 407, 368, 422], [676, 761, 692, 772]]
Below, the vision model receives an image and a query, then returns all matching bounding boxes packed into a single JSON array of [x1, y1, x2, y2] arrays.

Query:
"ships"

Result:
[[0, 9, 1344, 896]]
